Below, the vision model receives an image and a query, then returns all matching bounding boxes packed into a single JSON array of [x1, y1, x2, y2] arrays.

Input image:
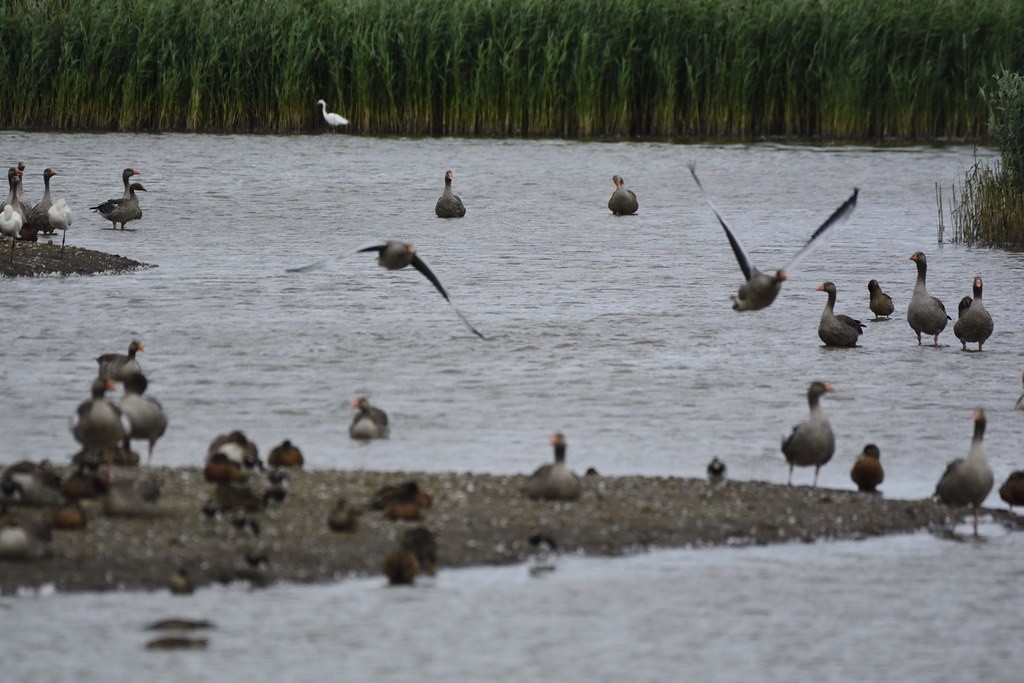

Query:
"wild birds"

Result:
[[816, 282, 867, 347], [868, 280, 894, 320], [0, 339, 1024, 584], [954, 276, 994, 351], [89, 168, 147, 230], [317, 99, 348, 133], [907, 251, 952, 345], [285, 241, 486, 339], [686, 160, 860, 311], [608, 175, 638, 215], [0, 161, 73, 263], [435, 170, 466, 218]]
